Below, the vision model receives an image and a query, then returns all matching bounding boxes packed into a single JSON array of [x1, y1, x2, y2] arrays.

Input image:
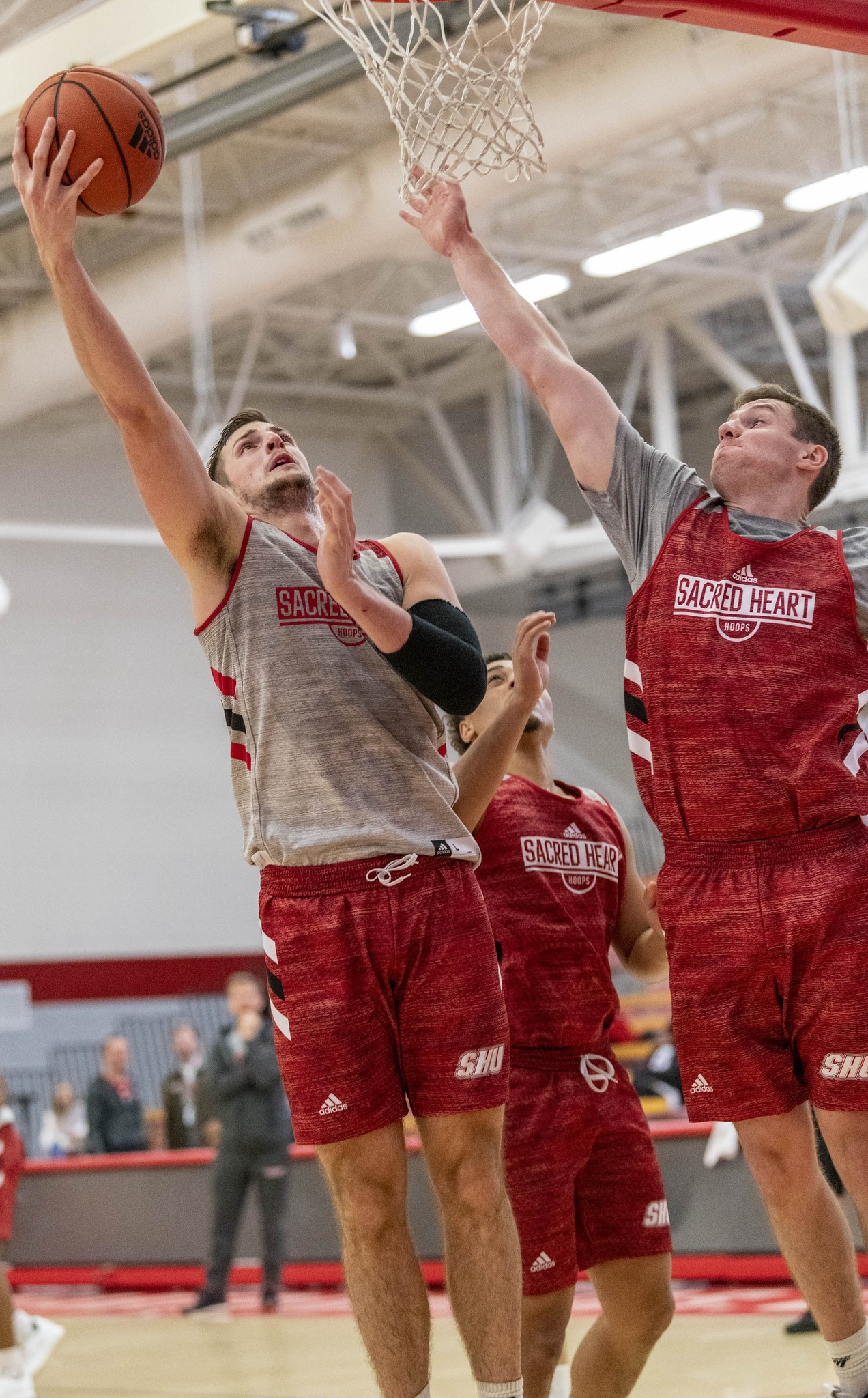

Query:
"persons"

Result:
[[404, 596, 696, 1398], [0, 1075, 22, 1347], [176, 970, 296, 1314], [781, 1100, 846, 1333], [382, 142, 868, 1398], [32, 1079, 92, 1163], [154, 1024, 220, 1148], [85, 1037, 152, 1154], [634, 1016, 681, 1108], [1, 109, 543, 1398]]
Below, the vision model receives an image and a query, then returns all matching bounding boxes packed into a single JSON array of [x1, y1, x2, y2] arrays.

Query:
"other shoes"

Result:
[[185, 1293, 230, 1316], [261, 1285, 280, 1311], [0, 1307, 64, 1398]]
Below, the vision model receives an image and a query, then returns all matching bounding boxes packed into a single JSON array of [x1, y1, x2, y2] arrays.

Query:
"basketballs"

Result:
[[18, 65, 166, 215]]
